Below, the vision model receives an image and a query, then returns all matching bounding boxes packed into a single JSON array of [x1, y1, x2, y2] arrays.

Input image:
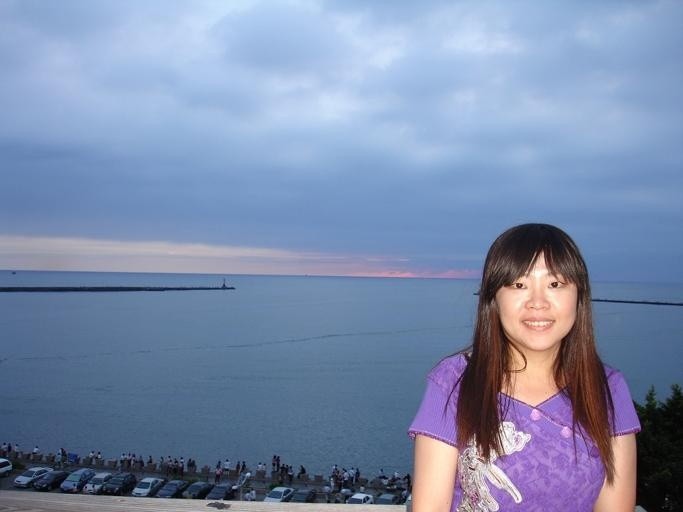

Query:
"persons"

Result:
[[407, 223, 642, 512]]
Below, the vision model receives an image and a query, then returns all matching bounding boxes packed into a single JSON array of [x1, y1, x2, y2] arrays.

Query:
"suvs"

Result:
[[0, 458, 11, 479]]
